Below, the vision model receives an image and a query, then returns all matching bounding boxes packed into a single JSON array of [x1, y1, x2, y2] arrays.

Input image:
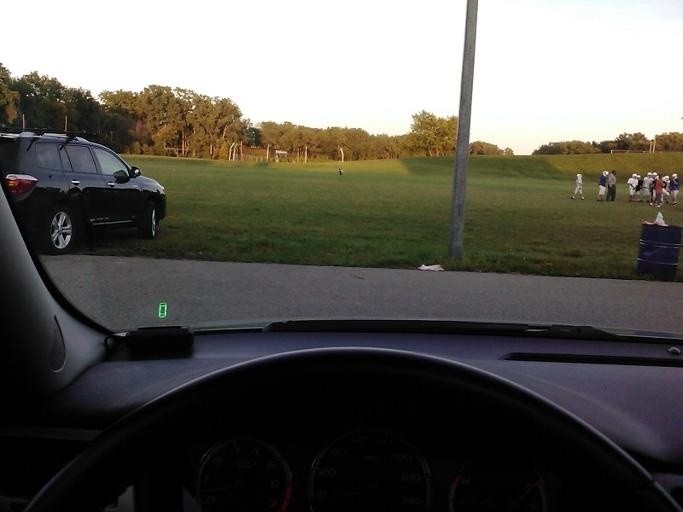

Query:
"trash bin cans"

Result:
[[637, 223, 681, 282]]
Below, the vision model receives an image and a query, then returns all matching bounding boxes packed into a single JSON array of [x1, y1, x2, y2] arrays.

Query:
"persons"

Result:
[[605, 170, 616, 201], [571, 169, 584, 200], [596, 171, 609, 201], [338, 167, 342, 175], [627, 171, 680, 207]]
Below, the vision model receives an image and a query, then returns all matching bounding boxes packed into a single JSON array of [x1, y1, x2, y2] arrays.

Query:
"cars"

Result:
[[1, 128, 167, 254]]
